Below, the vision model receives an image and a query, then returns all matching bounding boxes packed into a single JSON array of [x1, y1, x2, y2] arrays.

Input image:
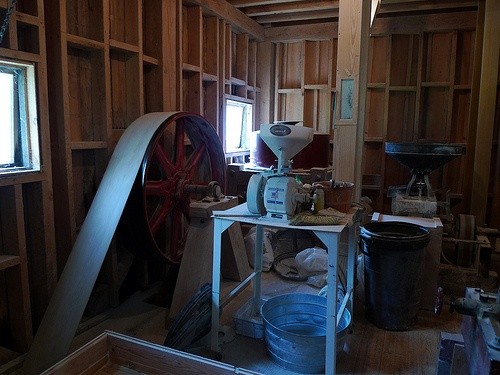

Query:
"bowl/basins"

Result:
[[259, 292, 352, 373]]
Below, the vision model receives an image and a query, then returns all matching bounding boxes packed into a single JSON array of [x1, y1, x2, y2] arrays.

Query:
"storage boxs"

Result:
[[37, 329, 267, 375], [232, 294, 271, 338]]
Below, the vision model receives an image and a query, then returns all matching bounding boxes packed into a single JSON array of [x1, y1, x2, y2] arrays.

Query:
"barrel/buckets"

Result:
[[360, 218, 430, 331]]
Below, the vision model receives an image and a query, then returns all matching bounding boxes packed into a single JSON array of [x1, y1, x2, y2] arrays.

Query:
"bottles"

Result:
[[314, 185, 324, 212]]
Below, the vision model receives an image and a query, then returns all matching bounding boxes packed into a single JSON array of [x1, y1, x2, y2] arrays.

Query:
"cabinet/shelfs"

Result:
[[0, 0, 258, 357], [362, 29, 480, 192], [274, 38, 334, 164]]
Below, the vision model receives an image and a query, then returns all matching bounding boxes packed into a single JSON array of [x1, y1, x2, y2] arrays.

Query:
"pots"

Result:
[[314, 180, 355, 212]]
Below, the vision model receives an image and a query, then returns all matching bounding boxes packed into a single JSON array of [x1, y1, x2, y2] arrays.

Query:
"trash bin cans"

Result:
[[360, 219, 431, 333]]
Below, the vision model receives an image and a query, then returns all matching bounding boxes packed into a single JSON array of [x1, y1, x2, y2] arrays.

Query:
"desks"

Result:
[[210, 202, 359, 375]]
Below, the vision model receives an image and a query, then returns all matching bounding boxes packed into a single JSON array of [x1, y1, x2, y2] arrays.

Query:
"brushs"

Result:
[[288, 214, 344, 226]]
